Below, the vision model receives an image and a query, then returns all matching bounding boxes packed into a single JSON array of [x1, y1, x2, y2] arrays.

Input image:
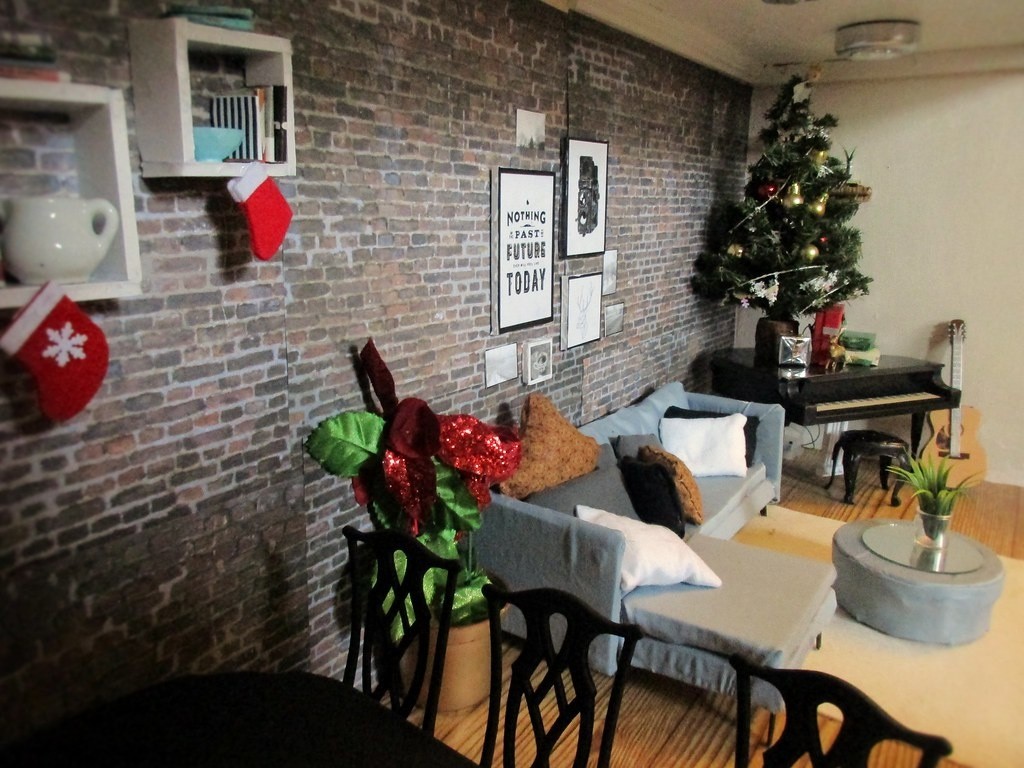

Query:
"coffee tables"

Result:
[[832, 517, 1005, 647]]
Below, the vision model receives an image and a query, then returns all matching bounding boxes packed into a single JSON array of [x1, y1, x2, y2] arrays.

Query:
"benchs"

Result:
[[470, 380, 785, 677]]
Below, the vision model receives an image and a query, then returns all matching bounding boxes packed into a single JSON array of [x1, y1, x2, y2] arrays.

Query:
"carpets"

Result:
[[704, 506, 1024, 768]]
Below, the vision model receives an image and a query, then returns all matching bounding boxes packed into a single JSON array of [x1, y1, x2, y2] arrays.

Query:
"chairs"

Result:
[[338, 525, 954, 768]]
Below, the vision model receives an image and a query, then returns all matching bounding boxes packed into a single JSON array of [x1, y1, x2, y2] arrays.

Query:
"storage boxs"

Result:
[[776, 333, 811, 367], [836, 329, 877, 366], [811, 304, 845, 366]]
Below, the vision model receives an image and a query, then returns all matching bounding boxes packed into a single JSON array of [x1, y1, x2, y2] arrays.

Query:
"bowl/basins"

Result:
[[192, 127, 245, 162]]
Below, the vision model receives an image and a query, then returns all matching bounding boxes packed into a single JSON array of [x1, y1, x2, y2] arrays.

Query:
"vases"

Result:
[[400, 574, 512, 713]]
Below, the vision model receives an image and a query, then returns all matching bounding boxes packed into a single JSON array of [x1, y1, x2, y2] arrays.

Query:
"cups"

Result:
[[1, 194, 119, 284]]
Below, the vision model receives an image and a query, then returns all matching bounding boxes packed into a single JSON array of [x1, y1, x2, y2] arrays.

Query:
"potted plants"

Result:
[[886, 444, 990, 550]]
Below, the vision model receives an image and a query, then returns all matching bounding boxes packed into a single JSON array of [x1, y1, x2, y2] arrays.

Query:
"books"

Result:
[[211, 81, 288, 164]]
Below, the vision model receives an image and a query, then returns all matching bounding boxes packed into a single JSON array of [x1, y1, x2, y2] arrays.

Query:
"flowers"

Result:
[[302, 335, 524, 636]]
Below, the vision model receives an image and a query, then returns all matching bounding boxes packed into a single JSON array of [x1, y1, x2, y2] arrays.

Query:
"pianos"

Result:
[[709, 343, 962, 519]]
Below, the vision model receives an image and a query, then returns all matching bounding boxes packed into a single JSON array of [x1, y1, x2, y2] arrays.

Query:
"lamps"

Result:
[[835, 20, 918, 62]]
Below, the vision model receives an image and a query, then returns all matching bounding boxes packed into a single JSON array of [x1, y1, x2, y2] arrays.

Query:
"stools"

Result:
[[623, 531, 838, 747], [824, 431, 908, 506]]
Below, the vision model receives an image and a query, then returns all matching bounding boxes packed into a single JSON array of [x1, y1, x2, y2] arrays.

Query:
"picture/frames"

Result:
[[558, 135, 608, 257]]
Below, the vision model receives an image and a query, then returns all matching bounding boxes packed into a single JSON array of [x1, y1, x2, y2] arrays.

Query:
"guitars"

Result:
[[918, 318, 988, 489]]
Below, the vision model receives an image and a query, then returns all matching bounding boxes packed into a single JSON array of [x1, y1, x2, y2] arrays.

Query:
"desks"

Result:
[[0, 668, 483, 767]]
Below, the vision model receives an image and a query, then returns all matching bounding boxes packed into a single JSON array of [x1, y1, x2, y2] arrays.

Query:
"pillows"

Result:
[[499, 392, 761, 587]]
